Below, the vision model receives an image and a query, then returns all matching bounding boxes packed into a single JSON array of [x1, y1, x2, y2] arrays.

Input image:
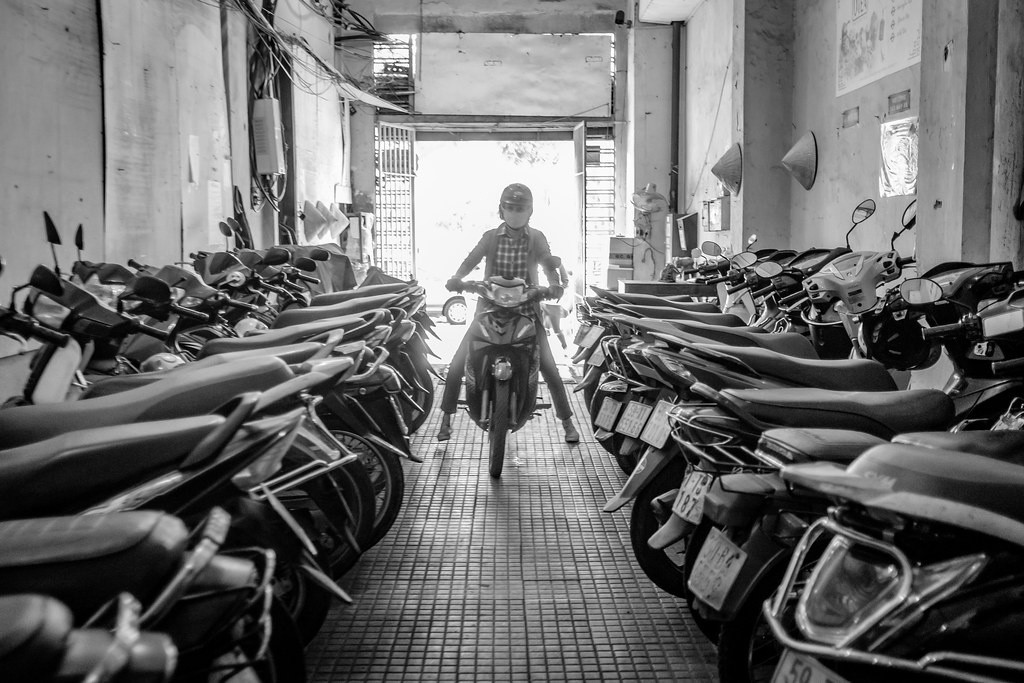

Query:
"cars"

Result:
[[414, 266, 481, 326]]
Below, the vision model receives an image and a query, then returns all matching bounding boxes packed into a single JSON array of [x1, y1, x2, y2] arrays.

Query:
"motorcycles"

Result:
[[568, 193, 1024, 683], [457, 255, 563, 479], [0, 188, 447, 683]]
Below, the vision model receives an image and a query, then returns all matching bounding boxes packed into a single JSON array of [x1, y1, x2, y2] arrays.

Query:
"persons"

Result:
[[537, 255, 569, 353], [436, 183, 578, 442]]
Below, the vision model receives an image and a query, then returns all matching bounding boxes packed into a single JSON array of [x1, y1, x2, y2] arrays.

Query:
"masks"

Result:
[[501, 206, 530, 229]]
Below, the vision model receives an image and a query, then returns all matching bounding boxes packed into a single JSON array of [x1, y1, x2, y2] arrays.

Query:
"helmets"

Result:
[[232, 318, 269, 337], [500, 183, 533, 206], [140, 353, 186, 373], [858, 307, 942, 372]]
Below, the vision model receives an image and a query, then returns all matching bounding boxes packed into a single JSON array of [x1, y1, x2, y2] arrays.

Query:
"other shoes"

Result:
[[437, 422, 453, 441], [562, 418, 579, 442]]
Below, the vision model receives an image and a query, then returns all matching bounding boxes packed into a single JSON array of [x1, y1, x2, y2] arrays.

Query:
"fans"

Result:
[[629, 183, 670, 216]]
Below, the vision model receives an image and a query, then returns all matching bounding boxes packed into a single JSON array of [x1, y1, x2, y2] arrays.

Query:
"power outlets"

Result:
[[643, 228, 650, 240]]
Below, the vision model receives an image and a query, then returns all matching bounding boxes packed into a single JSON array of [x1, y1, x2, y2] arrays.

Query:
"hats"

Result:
[[304, 200, 327, 242], [316, 201, 338, 240], [329, 203, 350, 239], [711, 143, 742, 197], [782, 131, 818, 190]]
